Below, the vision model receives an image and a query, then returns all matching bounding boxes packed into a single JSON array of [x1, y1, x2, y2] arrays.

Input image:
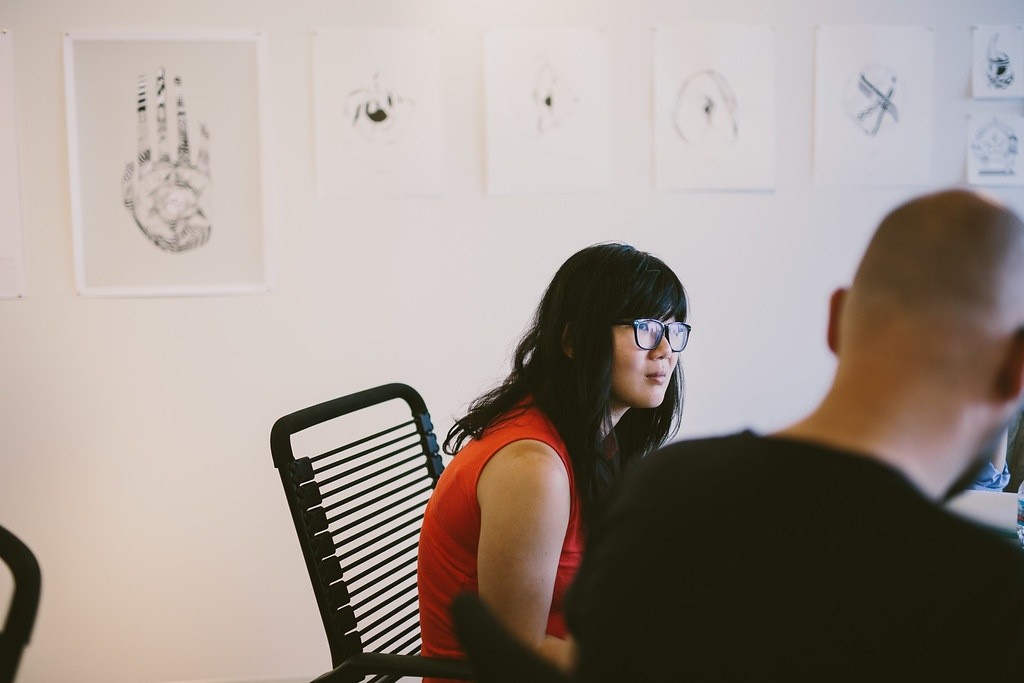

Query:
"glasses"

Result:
[[613, 318, 691, 352]]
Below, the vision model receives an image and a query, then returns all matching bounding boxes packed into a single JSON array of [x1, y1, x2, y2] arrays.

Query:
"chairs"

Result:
[[270, 382, 485, 682]]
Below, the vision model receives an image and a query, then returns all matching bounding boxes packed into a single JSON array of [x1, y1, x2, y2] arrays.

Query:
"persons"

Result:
[[418, 243, 688, 683], [964, 406, 1024, 493], [564, 188, 1024, 683]]
